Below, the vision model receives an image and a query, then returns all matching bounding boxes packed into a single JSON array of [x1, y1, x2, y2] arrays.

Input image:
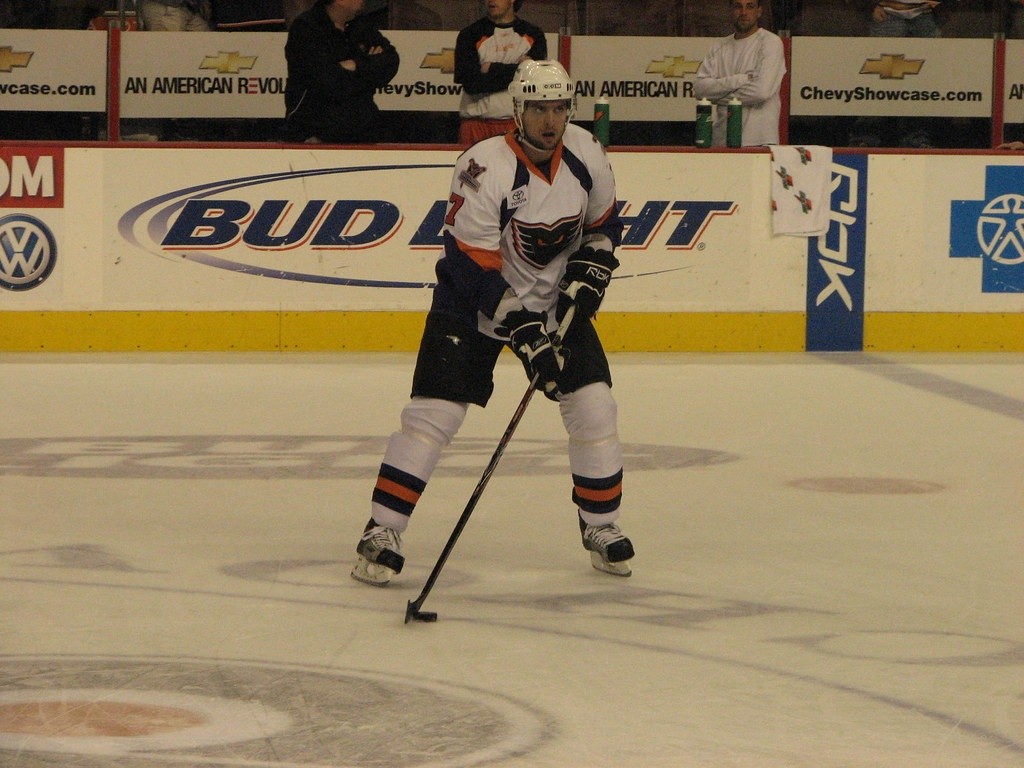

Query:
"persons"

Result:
[[284, 0, 400, 145], [142, 0, 212, 31], [351, 60, 636, 586], [695, 0, 786, 146], [849, 0, 943, 38], [454, 0, 548, 143]]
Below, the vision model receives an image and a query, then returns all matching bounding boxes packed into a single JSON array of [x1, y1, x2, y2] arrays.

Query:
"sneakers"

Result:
[[350, 518, 405, 586], [577, 509, 635, 576]]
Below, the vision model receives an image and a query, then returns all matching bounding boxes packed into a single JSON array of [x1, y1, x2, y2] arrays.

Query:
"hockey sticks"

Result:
[[403, 305, 576, 626]]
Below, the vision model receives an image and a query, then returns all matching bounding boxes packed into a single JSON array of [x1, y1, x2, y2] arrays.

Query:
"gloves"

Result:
[[555, 246, 620, 329], [508, 311, 572, 402]]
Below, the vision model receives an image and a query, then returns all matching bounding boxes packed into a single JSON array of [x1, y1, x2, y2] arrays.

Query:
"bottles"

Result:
[[593, 96, 609, 148], [726, 98, 743, 148], [694, 97, 713, 148]]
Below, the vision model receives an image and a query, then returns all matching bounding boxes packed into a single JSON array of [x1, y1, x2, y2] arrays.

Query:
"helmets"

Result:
[[508, 59, 574, 121]]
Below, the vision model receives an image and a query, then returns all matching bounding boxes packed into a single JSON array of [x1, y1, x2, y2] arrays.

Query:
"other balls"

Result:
[[412, 611, 438, 623]]
